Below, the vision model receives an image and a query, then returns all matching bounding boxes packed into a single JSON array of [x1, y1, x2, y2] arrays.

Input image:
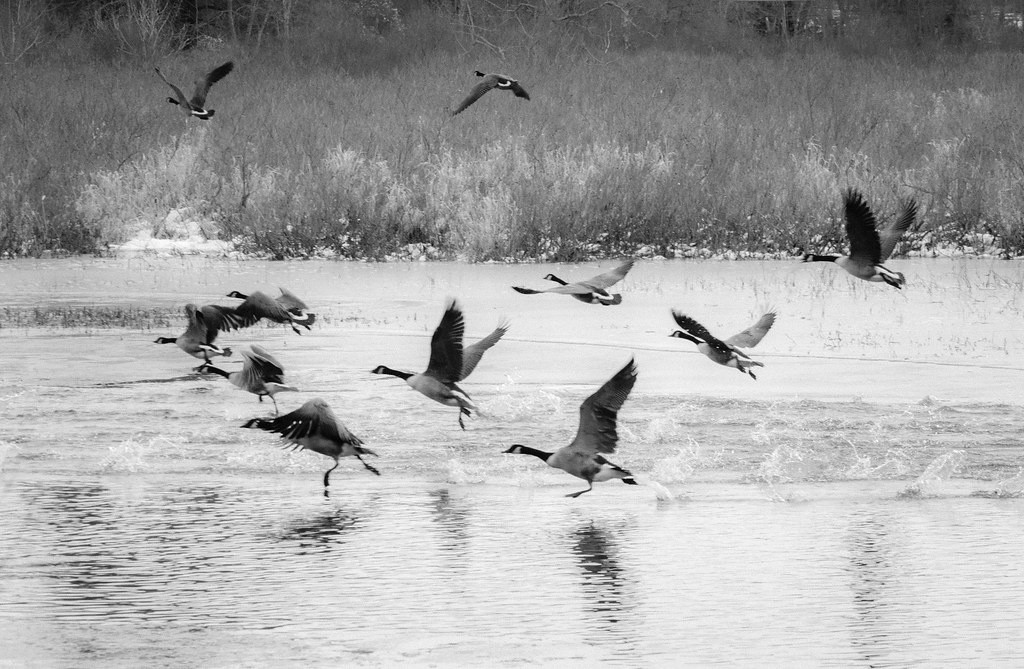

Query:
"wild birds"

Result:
[[451, 71, 531, 117], [152, 304, 232, 359], [227, 287, 316, 336], [239, 397, 379, 486], [802, 186, 919, 290], [666, 307, 778, 380], [197, 303, 242, 343], [194, 343, 298, 404], [154, 61, 234, 120], [369, 297, 510, 432], [501, 357, 639, 498], [510, 258, 635, 306]]
